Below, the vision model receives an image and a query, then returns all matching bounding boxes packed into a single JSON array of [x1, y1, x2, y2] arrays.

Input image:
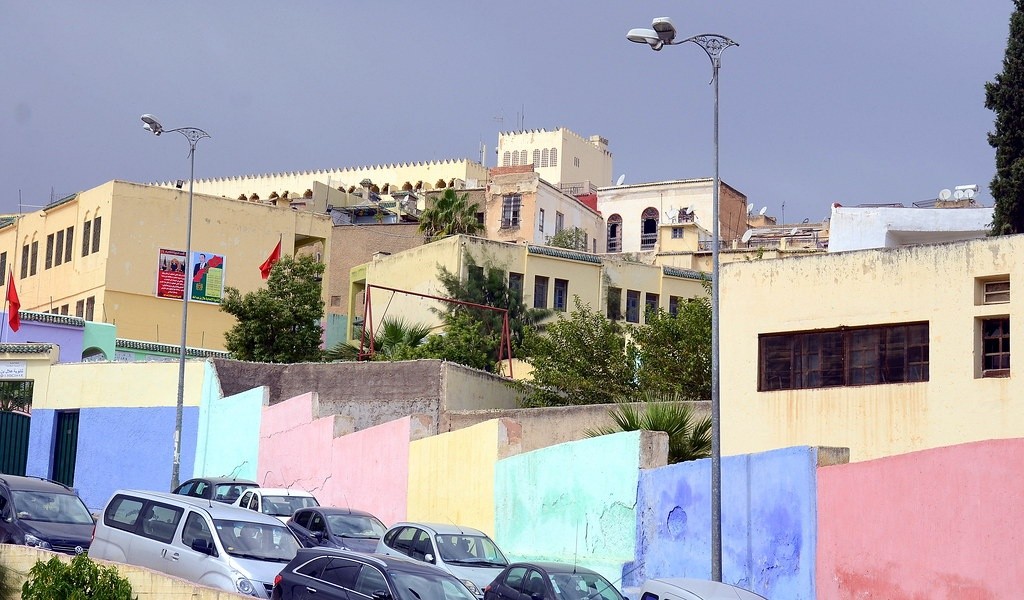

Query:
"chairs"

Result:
[[563, 580, 584, 600], [421, 538, 476, 559], [201, 487, 300, 514], [531, 577, 545, 594], [189, 522, 262, 552]]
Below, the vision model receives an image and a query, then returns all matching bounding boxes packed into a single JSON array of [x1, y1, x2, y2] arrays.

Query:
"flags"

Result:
[[259, 240, 281, 279], [6, 269, 21, 333]]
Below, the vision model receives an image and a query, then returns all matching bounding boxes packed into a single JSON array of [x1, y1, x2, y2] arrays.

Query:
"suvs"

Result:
[[0, 474, 97, 557], [271, 547, 478, 600]]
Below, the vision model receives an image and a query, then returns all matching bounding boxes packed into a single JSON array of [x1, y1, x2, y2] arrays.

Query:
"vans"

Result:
[[86, 490, 303, 600]]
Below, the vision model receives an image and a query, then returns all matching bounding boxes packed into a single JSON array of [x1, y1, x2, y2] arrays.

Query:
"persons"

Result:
[[193, 253, 207, 278], [161, 258, 185, 273]]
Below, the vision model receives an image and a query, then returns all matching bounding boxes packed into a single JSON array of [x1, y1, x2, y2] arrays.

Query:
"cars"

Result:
[[228, 488, 322, 534], [278, 507, 392, 556], [151, 477, 262, 520], [374, 522, 521, 600], [483, 562, 629, 600], [641, 579, 768, 600]]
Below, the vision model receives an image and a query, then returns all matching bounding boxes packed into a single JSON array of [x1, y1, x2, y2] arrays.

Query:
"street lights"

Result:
[[626, 16, 724, 582], [141, 113, 212, 493]]
